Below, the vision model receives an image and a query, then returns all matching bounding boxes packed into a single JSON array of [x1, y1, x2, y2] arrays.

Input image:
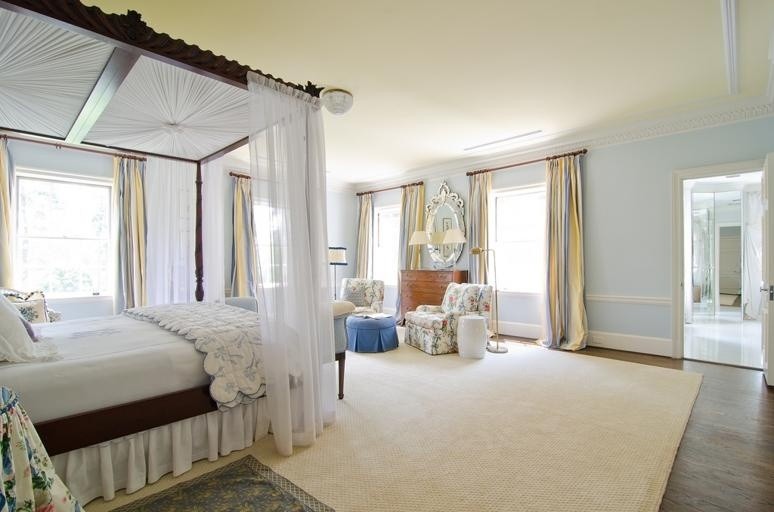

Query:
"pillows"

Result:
[[440, 282, 482, 313], [3, 291, 50, 324], [0, 293, 38, 363]]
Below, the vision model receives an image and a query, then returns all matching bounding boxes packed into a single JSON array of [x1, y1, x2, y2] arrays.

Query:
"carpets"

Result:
[[720, 293, 738, 305], [81, 324, 706, 512]]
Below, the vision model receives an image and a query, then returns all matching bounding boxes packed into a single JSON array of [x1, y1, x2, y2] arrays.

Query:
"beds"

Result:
[[0, 2, 324, 512]]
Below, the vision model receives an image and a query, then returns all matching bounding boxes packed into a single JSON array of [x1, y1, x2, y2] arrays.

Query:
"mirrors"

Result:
[[425, 182, 465, 268]]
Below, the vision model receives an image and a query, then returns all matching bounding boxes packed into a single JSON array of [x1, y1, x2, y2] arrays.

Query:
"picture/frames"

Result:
[[443, 218, 451, 256]]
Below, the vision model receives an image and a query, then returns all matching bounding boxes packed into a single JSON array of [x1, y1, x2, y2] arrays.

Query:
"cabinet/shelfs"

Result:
[[400, 270, 468, 327]]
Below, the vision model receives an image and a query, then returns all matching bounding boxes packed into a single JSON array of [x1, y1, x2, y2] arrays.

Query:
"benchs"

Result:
[[274, 301, 356, 400]]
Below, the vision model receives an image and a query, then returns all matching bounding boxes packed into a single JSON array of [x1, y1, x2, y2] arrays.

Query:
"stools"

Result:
[[345, 313, 399, 353], [458, 316, 489, 359]]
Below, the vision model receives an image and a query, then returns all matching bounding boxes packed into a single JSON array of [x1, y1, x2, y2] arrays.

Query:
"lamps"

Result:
[[409, 231, 430, 270], [470, 247, 508, 353], [328, 247, 347, 300], [321, 89, 354, 115], [430, 232, 444, 254], [442, 229, 466, 270]]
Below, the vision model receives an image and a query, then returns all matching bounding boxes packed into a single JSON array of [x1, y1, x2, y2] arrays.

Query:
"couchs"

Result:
[[405, 282, 493, 355], [340, 278, 384, 314]]
